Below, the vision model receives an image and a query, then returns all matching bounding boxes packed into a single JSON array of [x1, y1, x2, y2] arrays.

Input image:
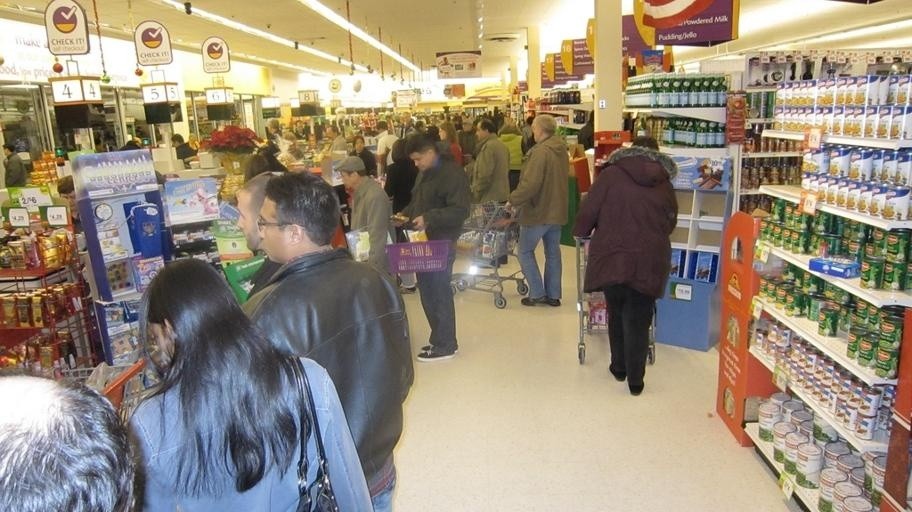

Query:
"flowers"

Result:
[[197, 124, 265, 153]]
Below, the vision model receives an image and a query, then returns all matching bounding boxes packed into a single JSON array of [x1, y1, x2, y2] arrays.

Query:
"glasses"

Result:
[[255, 215, 281, 229]]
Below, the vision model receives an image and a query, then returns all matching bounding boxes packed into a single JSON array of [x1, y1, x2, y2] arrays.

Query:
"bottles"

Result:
[[628, 57, 636, 76], [339, 204, 352, 231], [625, 73, 728, 108], [571, 111, 585, 124], [56, 146, 65, 166], [646, 119, 724, 149], [549, 87, 580, 103], [59, 339, 78, 370], [144, 138, 152, 149], [678, 65, 685, 74]]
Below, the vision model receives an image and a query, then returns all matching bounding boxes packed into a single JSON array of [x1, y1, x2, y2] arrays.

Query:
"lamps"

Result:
[[140, 66, 181, 104], [205, 73, 235, 107], [48, 55, 104, 107]]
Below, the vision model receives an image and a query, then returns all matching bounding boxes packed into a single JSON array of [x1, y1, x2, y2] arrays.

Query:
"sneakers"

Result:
[[399, 285, 417, 294], [421, 343, 459, 352], [609, 364, 626, 381], [521, 296, 547, 306], [547, 297, 561, 306], [629, 382, 644, 395], [416, 348, 455, 361]]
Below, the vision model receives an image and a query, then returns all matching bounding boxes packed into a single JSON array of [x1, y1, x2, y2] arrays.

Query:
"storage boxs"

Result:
[[153, 159, 185, 176], [197, 152, 218, 168], [594, 130, 630, 184], [152, 147, 177, 161]]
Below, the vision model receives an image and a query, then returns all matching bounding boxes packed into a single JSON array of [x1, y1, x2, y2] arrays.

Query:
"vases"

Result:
[[216, 153, 253, 202]]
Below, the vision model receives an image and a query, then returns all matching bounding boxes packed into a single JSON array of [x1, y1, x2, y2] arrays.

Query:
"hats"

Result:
[[266, 118, 279, 128], [333, 155, 365, 172]]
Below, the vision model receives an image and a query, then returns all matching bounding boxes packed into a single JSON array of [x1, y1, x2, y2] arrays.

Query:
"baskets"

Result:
[[386, 223, 452, 274]]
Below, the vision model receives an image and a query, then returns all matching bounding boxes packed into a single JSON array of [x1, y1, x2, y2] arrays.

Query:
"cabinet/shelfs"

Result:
[[716, 52, 912, 512], [536, 103, 594, 149], [622, 108, 733, 353], [0, 197, 99, 386]]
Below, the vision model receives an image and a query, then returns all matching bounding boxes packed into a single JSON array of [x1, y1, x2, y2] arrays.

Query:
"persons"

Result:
[[121, 137, 142, 151], [0, 375, 136, 512], [401, 110, 535, 193], [319, 125, 348, 206], [349, 135, 377, 176], [471, 119, 511, 269], [572, 135, 679, 395], [373, 122, 418, 214], [390, 133, 471, 361], [2, 143, 27, 187], [335, 156, 397, 282], [505, 114, 569, 307], [172, 134, 197, 159], [125, 258, 373, 512], [234, 171, 415, 512], [267, 119, 308, 159]]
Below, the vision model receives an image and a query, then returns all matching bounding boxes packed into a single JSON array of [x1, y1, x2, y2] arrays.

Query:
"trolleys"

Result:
[[40, 359, 151, 423], [574, 235, 658, 367], [444, 199, 531, 310]]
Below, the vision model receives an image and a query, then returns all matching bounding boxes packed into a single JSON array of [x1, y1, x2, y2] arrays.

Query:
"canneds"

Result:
[[758, 392, 887, 512], [741, 74, 912, 441]]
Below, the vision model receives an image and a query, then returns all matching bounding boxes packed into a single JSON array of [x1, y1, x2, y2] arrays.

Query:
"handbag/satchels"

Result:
[[288, 352, 338, 512]]
